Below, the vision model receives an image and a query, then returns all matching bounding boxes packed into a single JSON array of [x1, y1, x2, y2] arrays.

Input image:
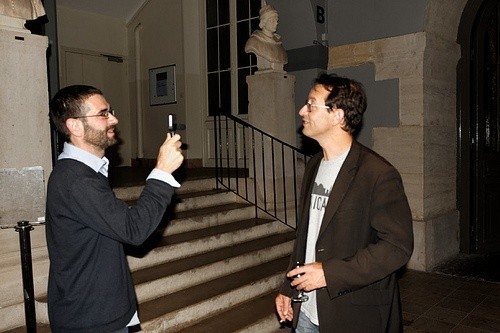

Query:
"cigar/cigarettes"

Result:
[[280, 315, 288, 323]]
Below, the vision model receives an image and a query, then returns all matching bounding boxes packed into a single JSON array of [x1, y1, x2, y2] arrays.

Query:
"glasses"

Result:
[[68, 108, 116, 120], [304, 99, 332, 113]]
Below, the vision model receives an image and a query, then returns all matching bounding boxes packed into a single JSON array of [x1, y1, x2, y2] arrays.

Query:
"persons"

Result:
[[0, 0, 45, 21], [275, 71, 414, 333], [45, 84, 185, 333], [244, 5, 289, 64]]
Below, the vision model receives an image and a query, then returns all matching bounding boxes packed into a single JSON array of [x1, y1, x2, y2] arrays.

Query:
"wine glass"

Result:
[[287, 257, 309, 302]]
